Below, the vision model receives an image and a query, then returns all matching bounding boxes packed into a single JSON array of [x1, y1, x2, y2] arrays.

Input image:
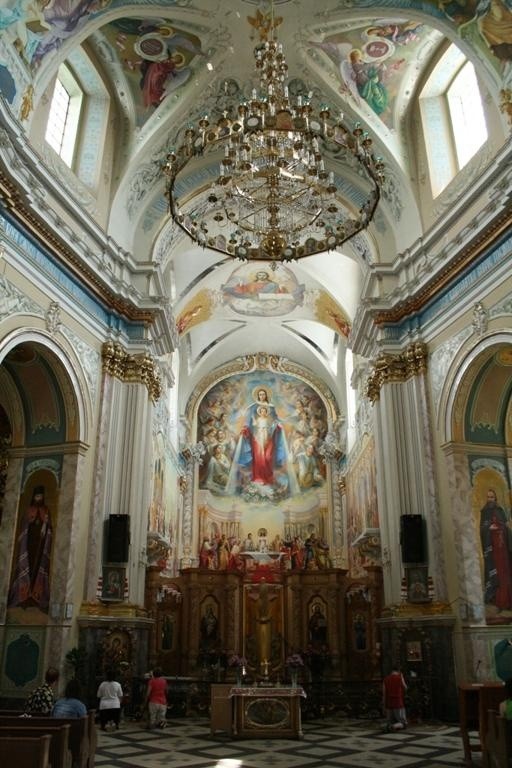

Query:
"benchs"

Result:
[[457, 681, 512, 768], [0, 696, 97, 768]]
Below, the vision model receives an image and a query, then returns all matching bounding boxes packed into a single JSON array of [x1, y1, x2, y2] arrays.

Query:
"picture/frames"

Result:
[[407, 567, 428, 602], [101, 566, 126, 602]]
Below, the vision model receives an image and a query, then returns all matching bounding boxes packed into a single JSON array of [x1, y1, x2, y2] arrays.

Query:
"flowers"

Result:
[[228, 654, 247, 666], [285, 653, 304, 666]]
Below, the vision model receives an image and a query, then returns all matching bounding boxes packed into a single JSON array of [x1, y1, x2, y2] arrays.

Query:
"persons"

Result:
[[248, 584, 276, 664], [307, 603, 326, 652], [195, 531, 328, 568], [478, 484, 511, 626], [237, 270, 289, 299], [22, 666, 60, 715], [407, 574, 428, 599], [288, 389, 324, 490], [353, 615, 366, 650], [380, 664, 409, 734], [49, 678, 88, 720], [12, 485, 52, 615], [201, 603, 218, 653], [345, 48, 392, 113], [108, 634, 123, 663], [128, 53, 186, 110], [105, 571, 119, 600], [405, 641, 419, 665], [144, 666, 167, 729], [251, 406, 273, 451], [200, 386, 236, 488], [162, 614, 173, 653], [95, 669, 123, 730], [241, 388, 283, 489]]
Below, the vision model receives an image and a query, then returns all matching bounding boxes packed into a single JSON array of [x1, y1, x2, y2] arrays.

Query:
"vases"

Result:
[[288, 664, 298, 688], [236, 665, 242, 687]]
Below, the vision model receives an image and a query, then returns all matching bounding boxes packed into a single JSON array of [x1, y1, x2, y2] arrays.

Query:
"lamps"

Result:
[[164, 1, 384, 271]]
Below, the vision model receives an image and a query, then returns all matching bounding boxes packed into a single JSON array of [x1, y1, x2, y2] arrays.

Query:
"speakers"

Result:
[[108, 514, 130, 561], [401, 514, 424, 562]]
